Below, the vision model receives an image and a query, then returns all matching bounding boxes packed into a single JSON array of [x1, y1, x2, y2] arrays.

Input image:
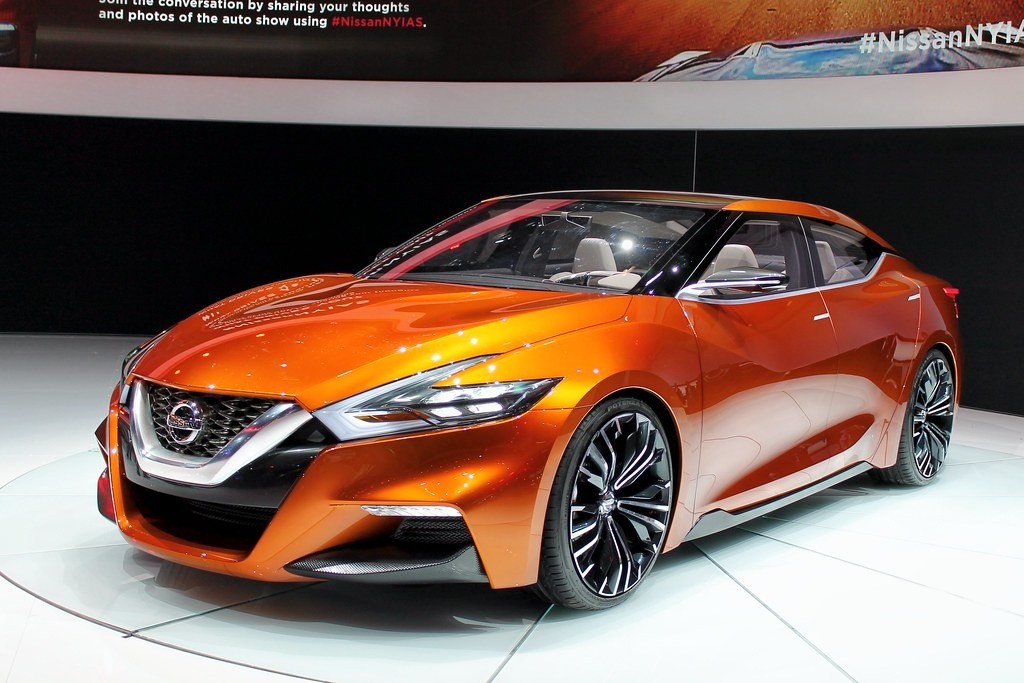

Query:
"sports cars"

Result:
[[95, 186, 965, 612]]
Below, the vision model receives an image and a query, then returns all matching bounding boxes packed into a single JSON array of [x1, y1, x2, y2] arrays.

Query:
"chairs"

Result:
[[571, 238, 617, 277], [710, 244, 758, 275]]
